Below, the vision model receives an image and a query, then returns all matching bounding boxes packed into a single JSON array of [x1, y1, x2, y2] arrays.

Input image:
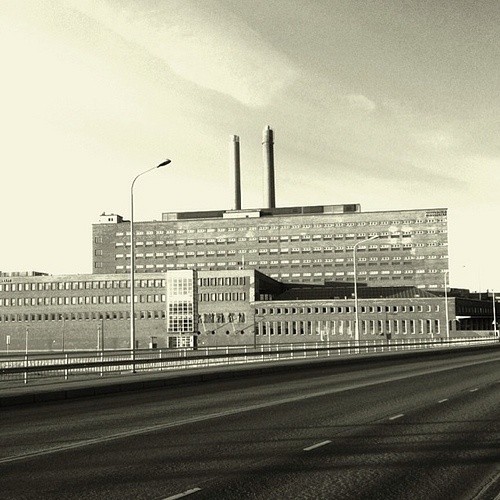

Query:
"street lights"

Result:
[[128, 158, 170, 370], [444, 265, 466, 338], [353, 234, 379, 341]]
